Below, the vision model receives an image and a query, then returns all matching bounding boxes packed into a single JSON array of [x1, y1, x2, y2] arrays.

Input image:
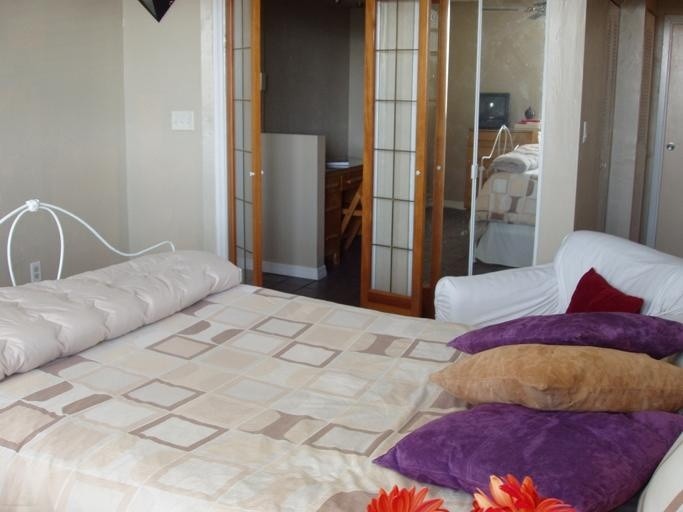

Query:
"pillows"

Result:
[[373, 268, 683, 510]]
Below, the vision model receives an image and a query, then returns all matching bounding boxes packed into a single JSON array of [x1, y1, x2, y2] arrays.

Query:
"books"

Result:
[[326, 159, 349, 166]]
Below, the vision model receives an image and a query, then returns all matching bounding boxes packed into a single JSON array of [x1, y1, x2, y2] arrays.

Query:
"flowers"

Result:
[[369, 464, 578, 510]]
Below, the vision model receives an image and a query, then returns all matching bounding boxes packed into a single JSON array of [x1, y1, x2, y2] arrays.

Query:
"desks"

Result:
[[315, 156, 362, 272], [463, 128, 541, 219]]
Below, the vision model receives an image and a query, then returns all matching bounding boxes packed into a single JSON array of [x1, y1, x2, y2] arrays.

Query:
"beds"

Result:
[[470, 120, 541, 274], [1, 197, 683, 510]]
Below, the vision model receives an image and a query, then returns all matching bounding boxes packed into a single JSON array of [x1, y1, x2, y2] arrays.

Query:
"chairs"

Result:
[[432, 225, 683, 332]]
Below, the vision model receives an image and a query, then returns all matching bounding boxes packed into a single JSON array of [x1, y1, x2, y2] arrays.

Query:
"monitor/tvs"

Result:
[[479, 92, 510, 129]]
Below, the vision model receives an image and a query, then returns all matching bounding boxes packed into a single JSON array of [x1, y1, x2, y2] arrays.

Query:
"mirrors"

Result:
[[422, 2, 543, 319]]
